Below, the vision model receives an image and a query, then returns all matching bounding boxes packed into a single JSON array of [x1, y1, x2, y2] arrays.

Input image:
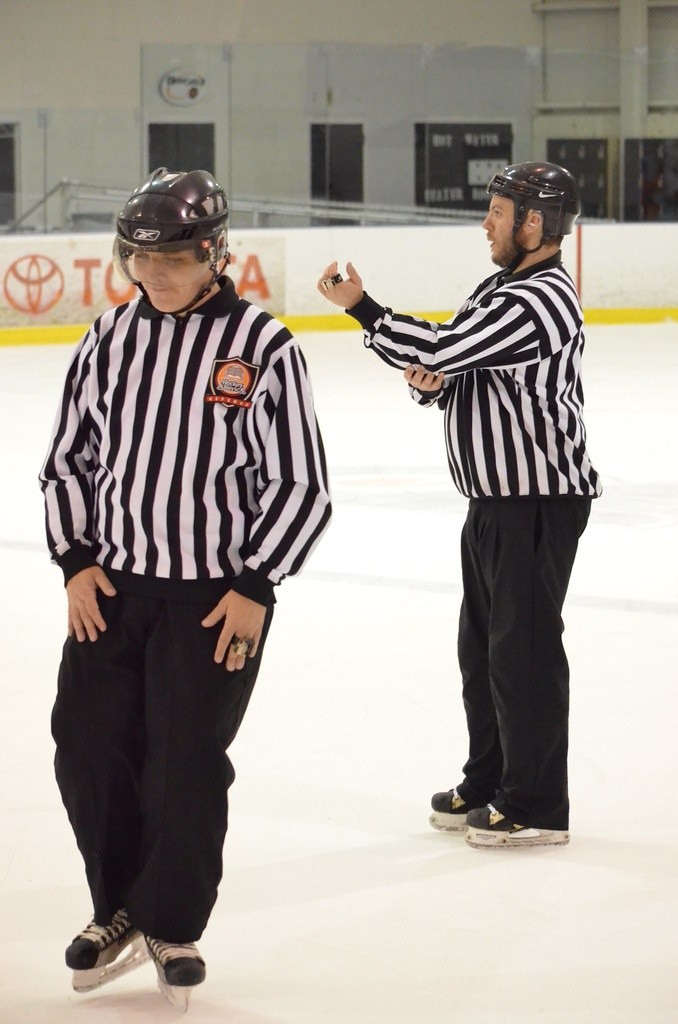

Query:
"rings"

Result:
[[233, 635, 253, 657]]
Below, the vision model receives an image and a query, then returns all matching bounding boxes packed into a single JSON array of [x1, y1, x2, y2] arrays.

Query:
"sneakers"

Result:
[[428, 788, 470, 833], [466, 802, 570, 847], [144, 935, 206, 1012], [65, 907, 151, 993]]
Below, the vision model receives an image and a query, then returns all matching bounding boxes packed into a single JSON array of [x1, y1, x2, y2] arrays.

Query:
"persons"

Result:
[[318, 161, 602, 850], [42, 166, 331, 1007]]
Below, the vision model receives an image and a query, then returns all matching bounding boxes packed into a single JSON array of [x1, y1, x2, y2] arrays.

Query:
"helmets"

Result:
[[117, 167, 228, 264], [485, 162, 581, 235]]
